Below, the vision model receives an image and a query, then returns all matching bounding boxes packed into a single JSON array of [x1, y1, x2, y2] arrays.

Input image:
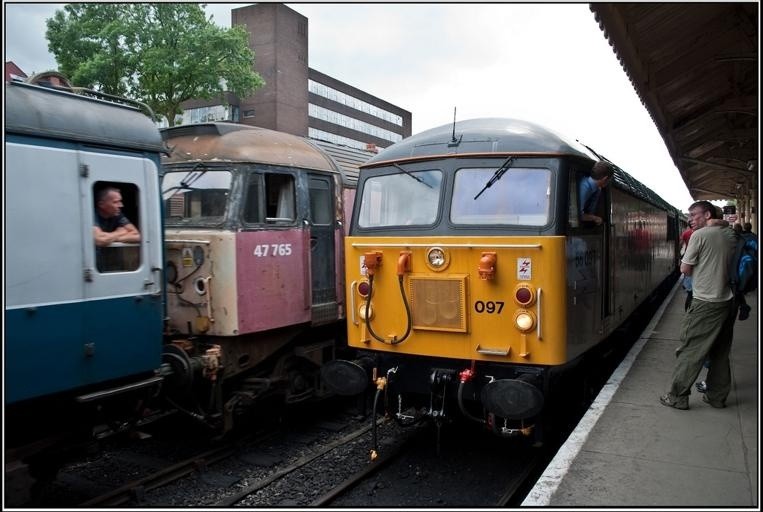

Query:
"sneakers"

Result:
[[703, 392, 725, 408], [696, 381, 707, 392], [660, 393, 689, 409], [739, 305, 750, 320]]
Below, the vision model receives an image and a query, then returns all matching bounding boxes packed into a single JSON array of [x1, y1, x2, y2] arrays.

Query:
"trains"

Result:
[[340, 114, 695, 462], [5, 69, 378, 450]]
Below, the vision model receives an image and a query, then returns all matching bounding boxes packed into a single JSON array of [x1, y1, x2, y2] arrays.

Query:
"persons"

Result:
[[657, 199, 738, 411], [622, 218, 651, 292], [677, 218, 757, 392], [703, 205, 752, 322], [578, 160, 615, 228], [91, 183, 141, 273]]
